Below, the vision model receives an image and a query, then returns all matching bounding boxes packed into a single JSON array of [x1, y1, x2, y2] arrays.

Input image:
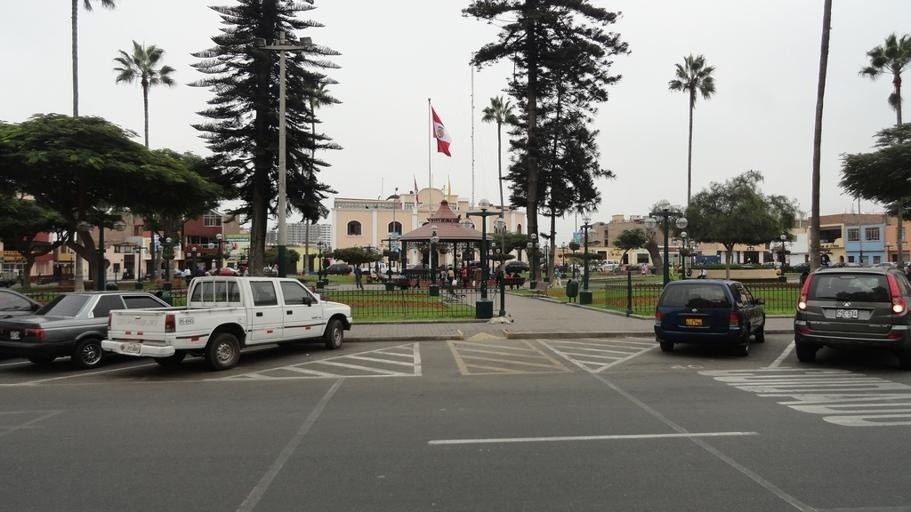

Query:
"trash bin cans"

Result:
[[155, 291, 173, 304], [565, 278, 579, 304]]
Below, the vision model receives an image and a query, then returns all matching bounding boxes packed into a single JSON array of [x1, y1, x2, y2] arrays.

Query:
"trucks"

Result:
[[694, 253, 723, 266], [0, 271, 18, 288]]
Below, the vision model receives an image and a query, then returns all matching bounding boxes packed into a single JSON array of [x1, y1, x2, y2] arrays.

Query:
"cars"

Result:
[[152, 265, 279, 280], [1, 288, 44, 356], [883, 262, 910, 276], [321, 259, 621, 276], [0, 290, 204, 369], [655, 279, 766, 356]]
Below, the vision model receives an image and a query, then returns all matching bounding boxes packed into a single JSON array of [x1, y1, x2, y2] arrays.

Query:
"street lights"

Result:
[[462, 198, 506, 320], [158, 237, 180, 281], [313, 236, 329, 281], [645, 199, 689, 290], [132, 240, 149, 283], [360, 215, 625, 290], [77, 201, 127, 291], [188, 247, 201, 278], [679, 231, 688, 279], [209, 233, 233, 276], [780, 232, 788, 283]]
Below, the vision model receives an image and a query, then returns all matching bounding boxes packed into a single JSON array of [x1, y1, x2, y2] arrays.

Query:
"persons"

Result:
[[171, 257, 652, 293]]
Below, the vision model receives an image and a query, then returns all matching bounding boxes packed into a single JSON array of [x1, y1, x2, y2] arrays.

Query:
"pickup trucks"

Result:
[[102, 275, 354, 371]]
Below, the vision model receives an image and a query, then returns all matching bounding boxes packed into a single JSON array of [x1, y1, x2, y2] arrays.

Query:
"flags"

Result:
[[447, 175, 452, 202], [429, 102, 453, 159], [413, 177, 424, 205]]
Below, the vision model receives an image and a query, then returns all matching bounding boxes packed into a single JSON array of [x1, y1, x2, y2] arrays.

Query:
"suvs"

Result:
[[794, 265, 911, 367]]
